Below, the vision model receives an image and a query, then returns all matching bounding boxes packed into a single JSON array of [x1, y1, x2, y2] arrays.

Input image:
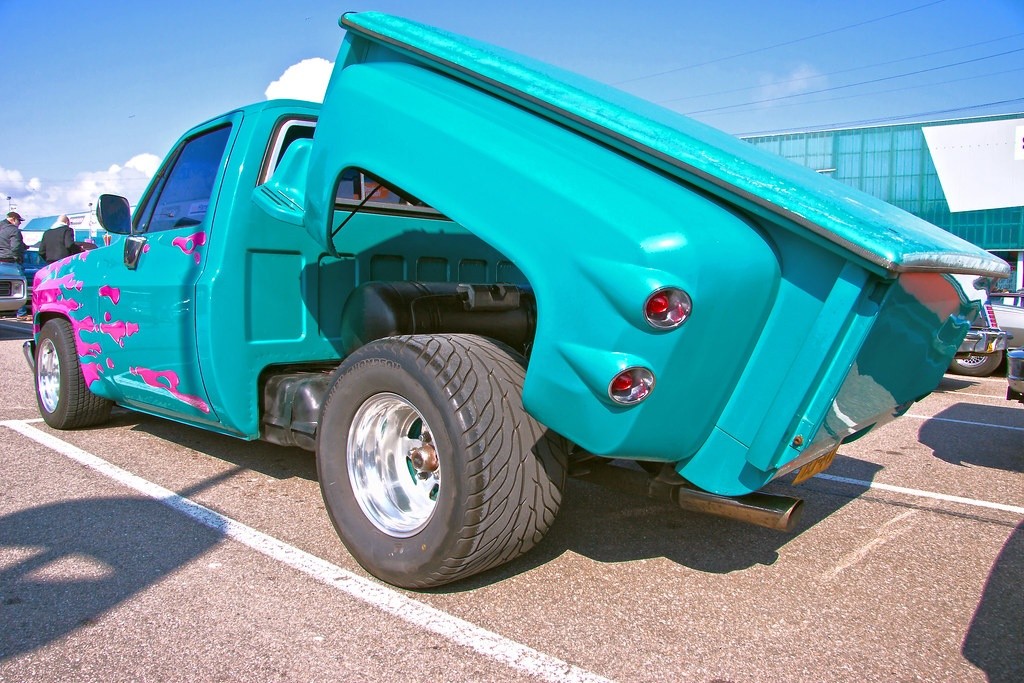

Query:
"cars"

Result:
[[0, 240, 99, 316], [951, 290, 1024, 409]]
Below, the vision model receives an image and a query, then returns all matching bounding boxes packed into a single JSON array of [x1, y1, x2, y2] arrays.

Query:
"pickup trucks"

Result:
[[17, 9, 1013, 593]]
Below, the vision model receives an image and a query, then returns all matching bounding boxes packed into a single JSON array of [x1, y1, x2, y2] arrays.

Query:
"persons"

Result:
[[38, 214, 87, 264], [0, 212, 33, 321]]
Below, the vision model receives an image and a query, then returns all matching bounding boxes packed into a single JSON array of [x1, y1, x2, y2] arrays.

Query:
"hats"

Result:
[[7, 212, 25, 221]]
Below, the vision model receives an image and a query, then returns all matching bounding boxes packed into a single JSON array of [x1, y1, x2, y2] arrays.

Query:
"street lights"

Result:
[[88, 203, 93, 239], [6, 196, 17, 212]]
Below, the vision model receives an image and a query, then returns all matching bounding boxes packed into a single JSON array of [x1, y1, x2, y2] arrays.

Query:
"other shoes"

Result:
[[16, 315, 33, 321]]
